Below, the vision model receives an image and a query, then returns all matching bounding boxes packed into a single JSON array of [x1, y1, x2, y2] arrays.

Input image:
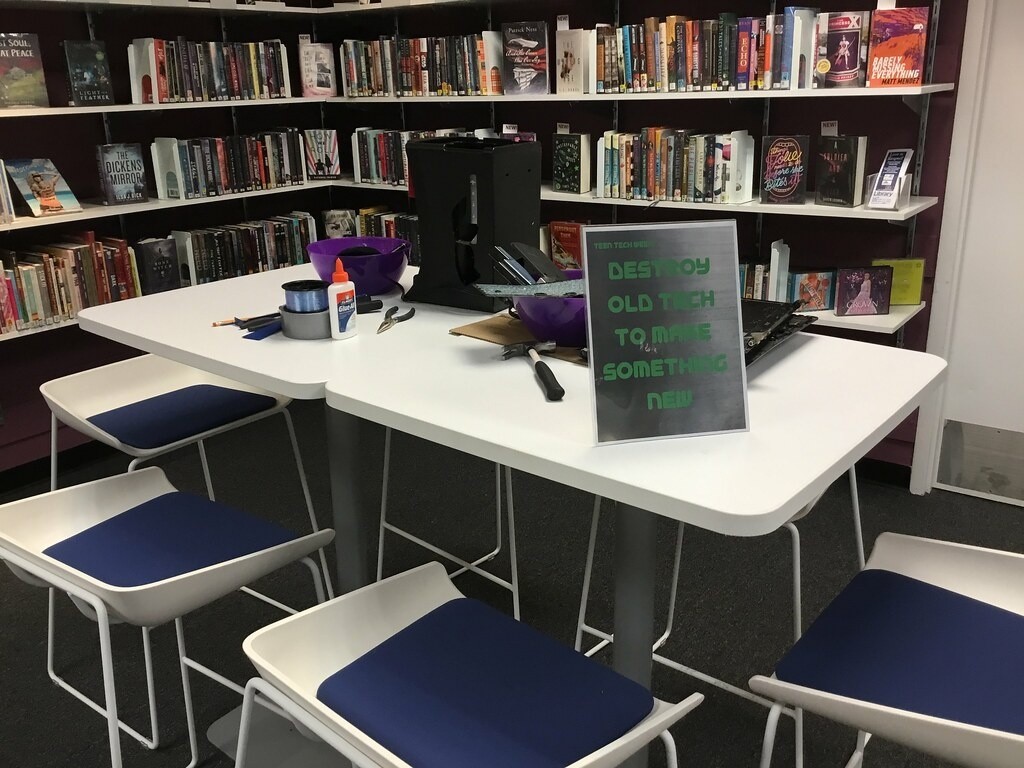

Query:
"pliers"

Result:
[[377, 306, 415, 335]]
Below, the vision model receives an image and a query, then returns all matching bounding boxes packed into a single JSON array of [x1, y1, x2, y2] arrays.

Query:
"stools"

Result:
[[231, 560, 705, 768], [38, 352, 337, 750], [0, 465, 336, 768], [748, 530, 1024, 768]]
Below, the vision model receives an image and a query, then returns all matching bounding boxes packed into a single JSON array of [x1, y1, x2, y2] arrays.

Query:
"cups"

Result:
[[282, 279, 330, 313]]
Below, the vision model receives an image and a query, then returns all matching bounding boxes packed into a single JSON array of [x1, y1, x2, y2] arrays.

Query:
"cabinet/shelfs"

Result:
[[0, 0, 998, 498]]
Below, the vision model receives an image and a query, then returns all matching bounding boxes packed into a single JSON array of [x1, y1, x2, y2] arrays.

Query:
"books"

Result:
[[0, 2, 929, 333]]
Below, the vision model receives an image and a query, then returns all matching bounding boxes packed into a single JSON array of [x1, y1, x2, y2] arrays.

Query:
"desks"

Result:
[[77, 260, 950, 768]]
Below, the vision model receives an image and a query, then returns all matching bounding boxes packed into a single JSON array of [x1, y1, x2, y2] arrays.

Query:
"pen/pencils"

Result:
[[213, 316, 254, 327]]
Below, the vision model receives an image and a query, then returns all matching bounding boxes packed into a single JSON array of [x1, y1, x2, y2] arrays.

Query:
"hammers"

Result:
[[497, 340, 566, 402]]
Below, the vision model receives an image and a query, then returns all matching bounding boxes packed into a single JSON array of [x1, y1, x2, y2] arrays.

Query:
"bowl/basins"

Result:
[[509, 270, 586, 348], [307, 236, 411, 295]]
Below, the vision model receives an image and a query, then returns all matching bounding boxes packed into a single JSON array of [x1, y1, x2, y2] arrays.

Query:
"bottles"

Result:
[[327, 258, 358, 339]]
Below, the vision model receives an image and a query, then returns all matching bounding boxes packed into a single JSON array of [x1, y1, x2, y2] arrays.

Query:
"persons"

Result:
[[314, 154, 333, 176], [30, 171, 65, 213], [317, 60, 329, 87], [70, 63, 110, 91], [917, 25, 922, 39], [836, 35, 850, 69], [560, 42, 576, 84], [885, 27, 889, 38], [799, 272, 829, 311], [845, 272, 877, 315]]
